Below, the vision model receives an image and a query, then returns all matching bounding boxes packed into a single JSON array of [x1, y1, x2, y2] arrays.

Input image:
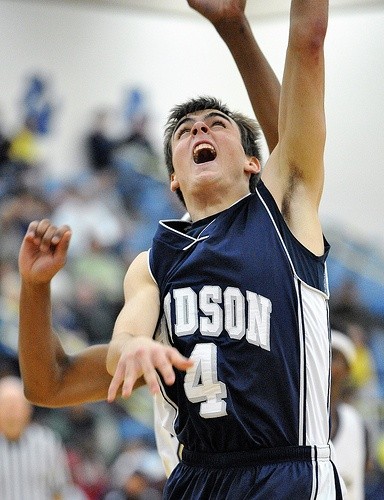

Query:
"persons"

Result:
[[3, 69, 384, 500], [103, 0, 350, 500], [16, 0, 339, 478]]
